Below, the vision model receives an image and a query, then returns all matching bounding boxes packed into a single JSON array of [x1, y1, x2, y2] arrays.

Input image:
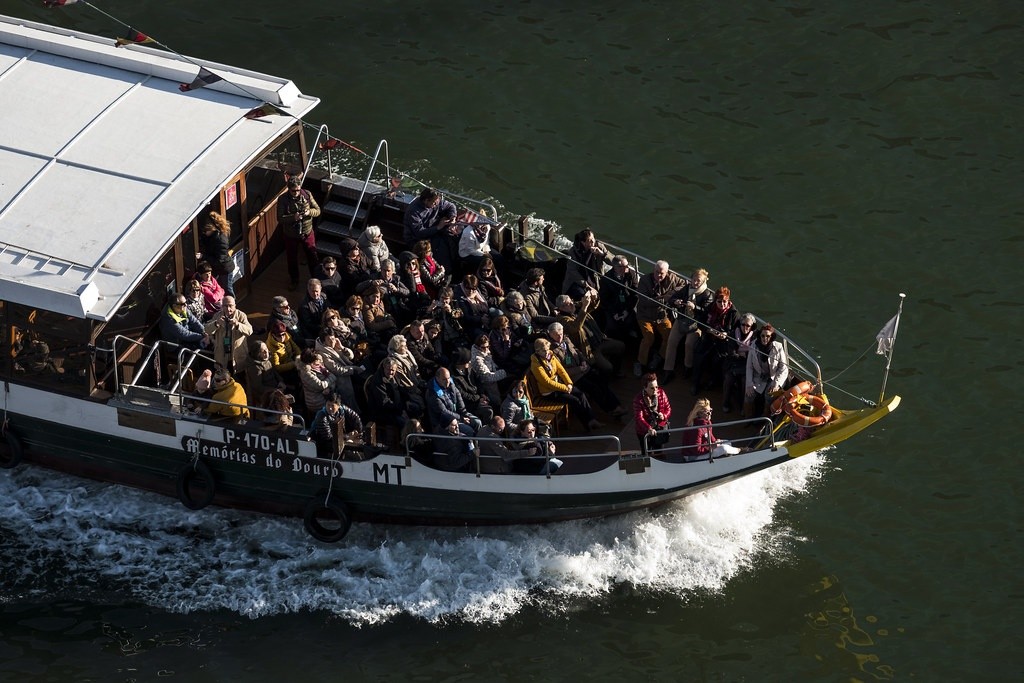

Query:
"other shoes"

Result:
[[602, 358, 767, 429], [584, 421, 606, 433]]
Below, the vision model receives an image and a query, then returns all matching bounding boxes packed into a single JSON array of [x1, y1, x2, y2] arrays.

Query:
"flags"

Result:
[[44, 0, 294, 117], [876, 314, 898, 355], [384, 174, 427, 195], [320, 138, 362, 153], [449, 208, 492, 234], [516, 238, 565, 263]]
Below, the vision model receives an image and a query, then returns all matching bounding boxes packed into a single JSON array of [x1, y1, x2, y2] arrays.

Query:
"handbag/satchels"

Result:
[[648, 425, 669, 446]]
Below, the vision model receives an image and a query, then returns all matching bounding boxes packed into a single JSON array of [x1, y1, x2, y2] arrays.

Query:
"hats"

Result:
[[440, 413, 456, 429], [195, 370, 212, 389], [339, 238, 357, 256]]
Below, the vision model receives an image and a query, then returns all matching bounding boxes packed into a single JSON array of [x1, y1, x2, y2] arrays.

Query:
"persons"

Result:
[[600, 255, 642, 346], [682, 399, 754, 460], [107, 272, 168, 329], [404, 188, 457, 244], [636, 259, 758, 417], [196, 211, 235, 298], [277, 175, 321, 290], [15, 340, 73, 383], [187, 370, 249, 418], [743, 325, 788, 426], [633, 373, 671, 455], [160, 262, 225, 359], [531, 338, 607, 434], [562, 230, 615, 300], [247, 224, 564, 472], [206, 296, 253, 373], [548, 322, 629, 419], [556, 290, 625, 375]]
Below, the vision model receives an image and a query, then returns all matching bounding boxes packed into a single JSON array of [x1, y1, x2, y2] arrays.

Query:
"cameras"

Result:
[[224, 317, 231, 322]]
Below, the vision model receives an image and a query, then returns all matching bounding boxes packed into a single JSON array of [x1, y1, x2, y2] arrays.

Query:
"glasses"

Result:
[[741, 323, 752, 327], [375, 233, 381, 239], [351, 306, 361, 311], [512, 389, 524, 394], [325, 314, 336, 322], [760, 334, 771, 338], [289, 188, 300, 192], [716, 300, 727, 303], [213, 377, 224, 383], [277, 305, 289, 309], [406, 262, 416, 266], [502, 326, 511, 331], [482, 268, 492, 273], [175, 300, 187, 306], [205, 230, 207, 233], [481, 345, 490, 349], [190, 289, 200, 292], [276, 333, 285, 338], [323, 267, 335, 271]]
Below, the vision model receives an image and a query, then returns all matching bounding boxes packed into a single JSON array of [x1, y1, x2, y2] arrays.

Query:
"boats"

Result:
[[0, 14, 904, 534]]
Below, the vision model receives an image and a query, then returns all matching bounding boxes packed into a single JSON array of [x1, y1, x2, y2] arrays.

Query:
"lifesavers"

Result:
[[177, 463, 217, 511], [304, 493, 352, 543], [785, 394, 832, 428], [770, 380, 815, 415]]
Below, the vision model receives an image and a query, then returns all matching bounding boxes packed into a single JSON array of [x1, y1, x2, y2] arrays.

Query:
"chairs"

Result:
[[167, 363, 198, 392], [520, 368, 569, 441]]
